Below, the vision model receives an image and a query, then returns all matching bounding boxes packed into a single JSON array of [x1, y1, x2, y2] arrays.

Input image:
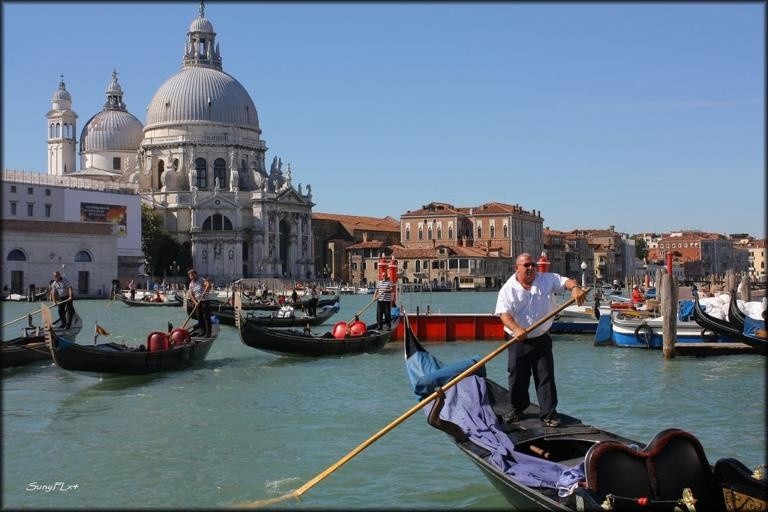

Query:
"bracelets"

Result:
[[572, 284, 582, 290]]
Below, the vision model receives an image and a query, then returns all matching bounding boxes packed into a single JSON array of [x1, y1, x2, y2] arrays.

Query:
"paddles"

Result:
[[234, 288, 591, 511]]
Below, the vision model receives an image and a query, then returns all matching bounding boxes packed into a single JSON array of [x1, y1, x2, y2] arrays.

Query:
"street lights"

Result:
[[581, 261, 587, 289], [170, 260, 180, 286]]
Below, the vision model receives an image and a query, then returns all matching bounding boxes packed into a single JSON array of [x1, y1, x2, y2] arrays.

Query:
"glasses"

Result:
[[516, 263, 536, 268]]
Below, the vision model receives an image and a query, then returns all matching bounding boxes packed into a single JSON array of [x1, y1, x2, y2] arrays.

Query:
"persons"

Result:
[[494, 253, 588, 429], [164, 281, 172, 295], [129, 279, 136, 301], [632, 283, 645, 310], [187, 269, 211, 338], [255, 280, 318, 317], [51, 270, 74, 330], [373, 271, 395, 331]]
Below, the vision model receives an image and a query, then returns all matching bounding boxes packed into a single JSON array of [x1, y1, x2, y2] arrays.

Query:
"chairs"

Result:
[[331, 321, 366, 339], [573, 428, 723, 511], [146, 328, 190, 352]]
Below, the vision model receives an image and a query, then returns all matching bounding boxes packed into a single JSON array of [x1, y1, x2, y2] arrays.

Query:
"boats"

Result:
[[551, 284, 767, 357], [401, 314, 767, 510]]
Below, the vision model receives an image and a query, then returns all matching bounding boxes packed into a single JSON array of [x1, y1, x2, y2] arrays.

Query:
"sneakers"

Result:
[[543, 417, 561, 428], [204, 332, 210, 338], [196, 331, 205, 337], [63, 325, 69, 329], [57, 325, 65, 328], [375, 326, 382, 330], [386, 327, 389, 331], [501, 408, 523, 422]]
